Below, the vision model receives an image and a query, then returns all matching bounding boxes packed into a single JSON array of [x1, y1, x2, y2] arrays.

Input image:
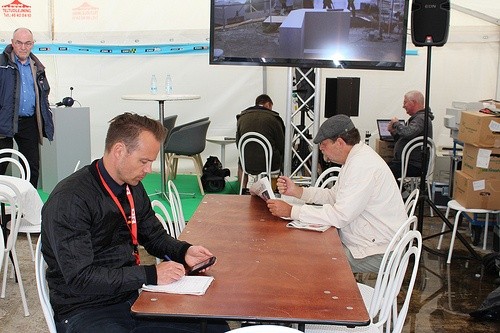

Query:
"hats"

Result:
[[313, 115, 355, 143]]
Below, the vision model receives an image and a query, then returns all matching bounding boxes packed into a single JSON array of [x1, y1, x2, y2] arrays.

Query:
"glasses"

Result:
[[14, 41, 33, 47]]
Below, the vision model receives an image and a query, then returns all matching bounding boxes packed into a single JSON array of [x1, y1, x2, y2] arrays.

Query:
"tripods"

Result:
[[406, 47, 480, 261]]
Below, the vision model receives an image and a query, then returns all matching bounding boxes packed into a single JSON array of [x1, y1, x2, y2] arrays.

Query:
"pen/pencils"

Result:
[[164, 255, 171, 261]]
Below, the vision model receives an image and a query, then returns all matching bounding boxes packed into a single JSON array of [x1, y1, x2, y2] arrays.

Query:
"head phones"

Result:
[[56, 97, 74, 106]]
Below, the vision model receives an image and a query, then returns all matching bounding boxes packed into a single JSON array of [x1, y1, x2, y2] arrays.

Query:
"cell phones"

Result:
[[188, 256, 216, 275]]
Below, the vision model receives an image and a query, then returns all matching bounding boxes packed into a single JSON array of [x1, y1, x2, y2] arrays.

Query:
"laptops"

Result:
[[377, 120, 406, 142]]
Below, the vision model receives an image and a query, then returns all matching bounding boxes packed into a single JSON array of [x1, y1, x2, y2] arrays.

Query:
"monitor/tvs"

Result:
[[211, 0, 409, 70]]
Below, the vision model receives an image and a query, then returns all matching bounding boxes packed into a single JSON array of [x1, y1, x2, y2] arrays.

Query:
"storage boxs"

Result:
[[452, 111, 500, 210]]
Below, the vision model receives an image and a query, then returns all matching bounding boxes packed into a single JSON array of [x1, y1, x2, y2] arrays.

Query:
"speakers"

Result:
[[411, 0, 451, 46], [325, 77, 360, 117]]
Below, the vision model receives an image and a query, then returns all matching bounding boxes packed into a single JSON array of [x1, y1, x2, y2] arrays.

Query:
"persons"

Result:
[[235, 93, 287, 194], [267, 113, 409, 274], [41, 111, 231, 333], [0, 27, 55, 188], [388, 91, 435, 179]]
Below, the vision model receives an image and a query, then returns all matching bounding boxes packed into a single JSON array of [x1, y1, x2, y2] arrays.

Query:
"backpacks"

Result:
[[200, 156, 231, 192]]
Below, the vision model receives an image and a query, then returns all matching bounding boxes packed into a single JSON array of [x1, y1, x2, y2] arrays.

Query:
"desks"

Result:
[[121, 94, 202, 208], [129, 194, 370, 332], [206, 135, 237, 182]]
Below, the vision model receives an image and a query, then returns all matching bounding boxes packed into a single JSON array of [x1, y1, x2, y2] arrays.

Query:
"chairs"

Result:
[[437, 199, 500, 264], [225, 131, 434, 333], [0, 149, 186, 333], [153, 115, 211, 196]]
